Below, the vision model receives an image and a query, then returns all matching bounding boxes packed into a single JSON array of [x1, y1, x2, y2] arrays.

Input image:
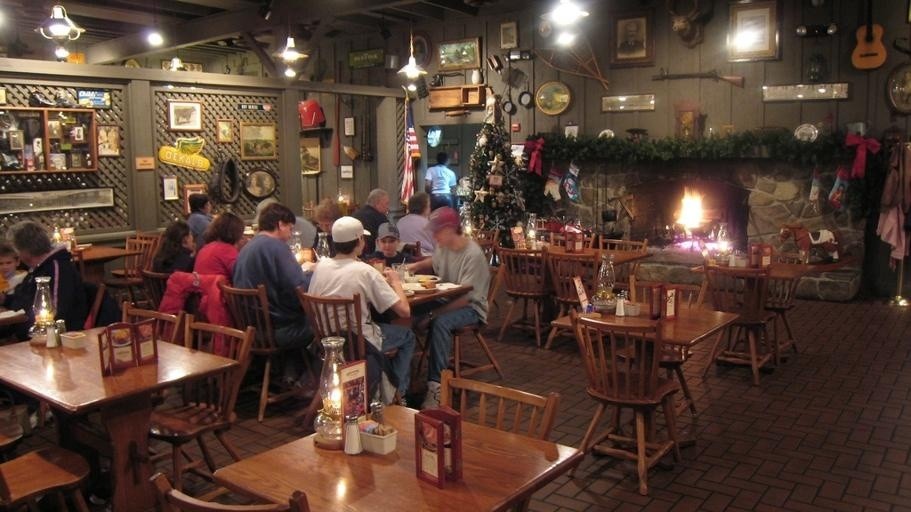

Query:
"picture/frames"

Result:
[[0, 87, 7, 106], [162, 59, 204, 73], [161, 98, 277, 217], [726, 0, 785, 64], [97, 124, 121, 159], [500, 20, 519, 51], [609, 11, 656, 69], [77, 86, 114, 112], [433, 37, 483, 71], [674, 102, 699, 144]]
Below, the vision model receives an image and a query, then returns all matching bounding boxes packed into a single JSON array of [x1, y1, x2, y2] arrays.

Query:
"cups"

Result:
[[391, 263, 404, 282]]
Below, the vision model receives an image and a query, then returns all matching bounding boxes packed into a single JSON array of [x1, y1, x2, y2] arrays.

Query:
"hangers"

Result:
[[397, 20, 428, 94], [883, 129, 911, 307]]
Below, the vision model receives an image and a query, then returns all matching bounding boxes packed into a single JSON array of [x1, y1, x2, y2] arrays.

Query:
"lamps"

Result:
[[33, 2, 86, 61], [272, 1, 310, 80], [170, 2, 185, 71], [145, 0, 167, 49], [541, 0, 591, 50]]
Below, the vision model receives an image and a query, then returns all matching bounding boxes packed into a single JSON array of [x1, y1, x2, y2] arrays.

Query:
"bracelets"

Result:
[[427, 309, 433, 321]]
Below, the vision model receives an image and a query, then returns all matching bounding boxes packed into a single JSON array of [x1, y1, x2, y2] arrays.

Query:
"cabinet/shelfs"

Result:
[[428, 83, 486, 111], [1, 105, 99, 177]]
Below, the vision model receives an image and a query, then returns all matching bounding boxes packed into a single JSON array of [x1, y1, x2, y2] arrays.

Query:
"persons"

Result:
[[193, 213, 245, 277], [0, 245, 28, 294], [307, 215, 415, 408], [188, 151, 457, 268], [152, 220, 195, 314], [0, 221, 87, 332], [232, 203, 323, 400], [404, 206, 491, 410]]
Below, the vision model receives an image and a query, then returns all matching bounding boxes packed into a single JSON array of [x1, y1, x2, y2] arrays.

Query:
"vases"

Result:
[[471, 69, 482, 84]]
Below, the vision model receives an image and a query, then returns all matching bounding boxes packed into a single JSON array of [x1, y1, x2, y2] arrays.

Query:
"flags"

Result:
[[400, 101, 421, 214]]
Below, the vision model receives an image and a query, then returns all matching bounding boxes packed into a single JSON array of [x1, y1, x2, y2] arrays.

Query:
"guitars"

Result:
[[851, 0, 887, 70]]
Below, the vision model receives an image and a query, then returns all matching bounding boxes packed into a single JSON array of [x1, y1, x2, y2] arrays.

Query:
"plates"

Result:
[[399, 275, 440, 296]]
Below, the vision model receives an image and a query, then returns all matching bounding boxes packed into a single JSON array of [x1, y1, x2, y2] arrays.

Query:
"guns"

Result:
[[651, 73, 744, 89]]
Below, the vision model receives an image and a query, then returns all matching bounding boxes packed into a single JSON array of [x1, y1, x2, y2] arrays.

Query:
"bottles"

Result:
[[369, 402, 383, 426], [615, 294, 624, 316], [55, 320, 65, 333], [0, 172, 86, 192], [620, 290, 628, 300], [343, 415, 362, 455], [45, 322, 57, 348]]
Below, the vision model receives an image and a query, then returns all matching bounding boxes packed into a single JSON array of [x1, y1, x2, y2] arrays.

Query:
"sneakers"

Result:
[[421, 387, 441, 409]]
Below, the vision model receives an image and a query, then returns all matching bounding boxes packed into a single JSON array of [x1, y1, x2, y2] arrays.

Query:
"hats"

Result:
[[422, 206, 460, 230], [378, 222, 400, 240], [331, 216, 371, 242]]
